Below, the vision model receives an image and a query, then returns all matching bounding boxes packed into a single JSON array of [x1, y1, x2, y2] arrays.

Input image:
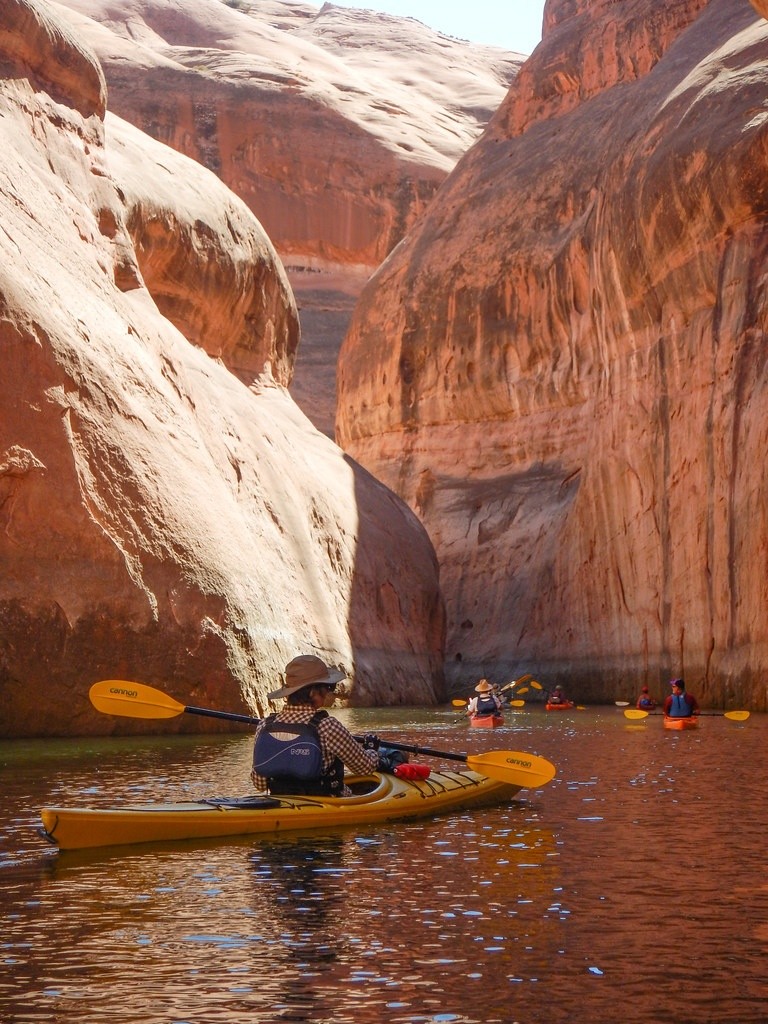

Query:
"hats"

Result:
[[556, 686, 562, 690], [267, 655, 347, 699], [670, 679, 685, 688], [642, 686, 650, 692], [475, 679, 493, 691]]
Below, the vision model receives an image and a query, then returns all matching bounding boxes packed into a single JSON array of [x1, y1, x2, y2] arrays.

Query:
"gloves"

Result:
[[361, 733, 381, 751]]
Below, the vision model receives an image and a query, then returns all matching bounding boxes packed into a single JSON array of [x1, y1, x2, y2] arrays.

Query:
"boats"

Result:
[[663, 716, 700, 732], [470, 714, 506, 729], [546, 704, 573, 711], [40, 771, 524, 850]]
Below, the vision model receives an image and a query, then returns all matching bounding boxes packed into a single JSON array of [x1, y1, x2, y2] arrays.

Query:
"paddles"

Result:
[[500, 680, 516, 691], [530, 680, 586, 710], [452, 699, 526, 707], [87, 678, 557, 790], [615, 701, 663, 707], [623, 709, 751, 721], [451, 674, 532, 724], [516, 688, 529, 695]]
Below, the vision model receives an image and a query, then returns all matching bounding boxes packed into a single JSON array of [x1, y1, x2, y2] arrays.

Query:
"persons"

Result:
[[550, 685, 563, 704], [250, 655, 381, 797], [466, 679, 506, 716], [636, 687, 656, 709], [662, 679, 700, 717]]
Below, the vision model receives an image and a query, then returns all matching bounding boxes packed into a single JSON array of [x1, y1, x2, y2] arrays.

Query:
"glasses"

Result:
[[313, 683, 338, 692]]
